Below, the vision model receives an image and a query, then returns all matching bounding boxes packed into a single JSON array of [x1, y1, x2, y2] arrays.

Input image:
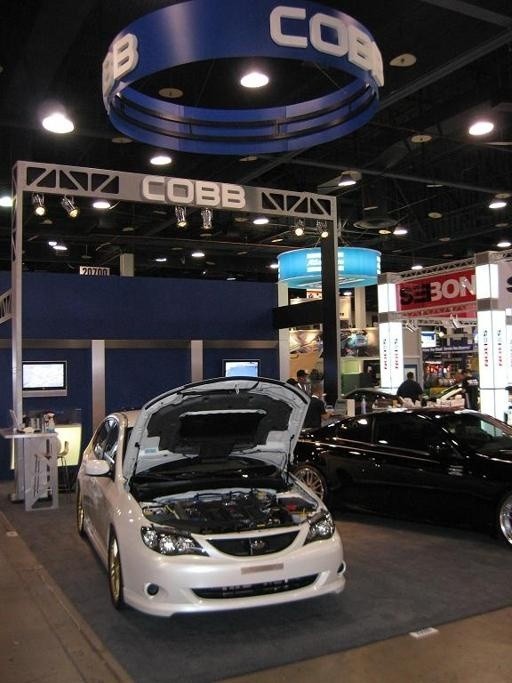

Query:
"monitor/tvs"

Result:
[[23, 360, 68, 398], [222, 358, 261, 378]]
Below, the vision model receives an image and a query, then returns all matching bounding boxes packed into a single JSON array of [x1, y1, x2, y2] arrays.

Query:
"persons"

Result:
[[296, 369, 310, 395], [397, 371, 424, 406], [304, 382, 333, 428]]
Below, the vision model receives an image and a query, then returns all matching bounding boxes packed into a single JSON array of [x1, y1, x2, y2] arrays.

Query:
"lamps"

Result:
[[339, 172, 358, 186], [61, 194, 78, 218], [496, 236, 512, 249], [53, 240, 68, 251], [175, 205, 186, 227], [488, 194, 509, 211], [201, 208, 214, 229], [468, 117, 495, 139], [292, 222, 306, 238], [31, 191, 46, 216], [251, 214, 271, 228], [267, 260, 280, 270], [150, 150, 172, 166], [92, 198, 111, 209], [390, 224, 409, 237], [315, 220, 330, 238], [410, 261, 424, 270]]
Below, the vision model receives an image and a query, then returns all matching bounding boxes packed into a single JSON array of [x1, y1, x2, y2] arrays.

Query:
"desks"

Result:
[[0, 428, 59, 512], [10, 423, 82, 493]]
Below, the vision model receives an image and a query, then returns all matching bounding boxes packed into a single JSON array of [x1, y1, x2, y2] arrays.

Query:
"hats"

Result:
[[286, 369, 309, 385]]
